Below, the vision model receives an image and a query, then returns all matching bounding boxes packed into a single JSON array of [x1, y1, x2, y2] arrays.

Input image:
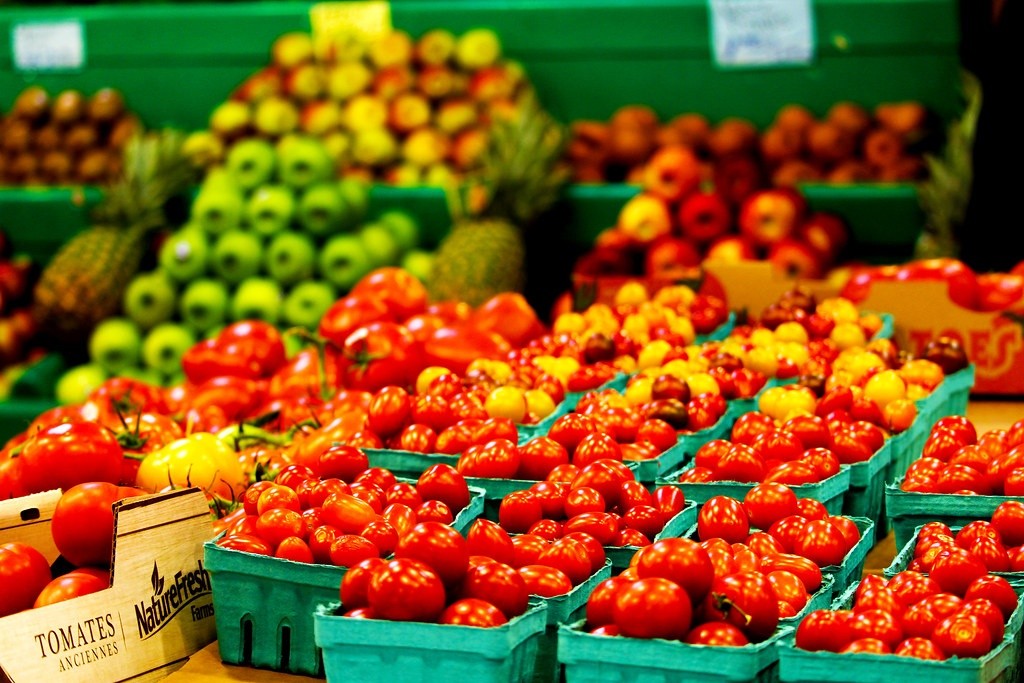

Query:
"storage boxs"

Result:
[[363, 312, 1024, 594], [0, 487, 216, 683], [557, 619, 796, 683], [777, 580, 1024, 683], [204, 476, 486, 680], [529, 558, 613, 683], [313, 600, 548, 683]]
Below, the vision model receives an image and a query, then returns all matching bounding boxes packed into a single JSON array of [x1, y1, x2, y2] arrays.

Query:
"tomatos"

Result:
[[0, 267, 1024, 660]]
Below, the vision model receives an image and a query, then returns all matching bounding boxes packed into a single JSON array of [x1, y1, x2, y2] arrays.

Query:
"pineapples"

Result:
[[32, 174, 179, 330]]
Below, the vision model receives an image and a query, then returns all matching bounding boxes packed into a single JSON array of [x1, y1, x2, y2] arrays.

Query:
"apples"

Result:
[[50, 23, 532, 403], [558, 149, 845, 316]]
